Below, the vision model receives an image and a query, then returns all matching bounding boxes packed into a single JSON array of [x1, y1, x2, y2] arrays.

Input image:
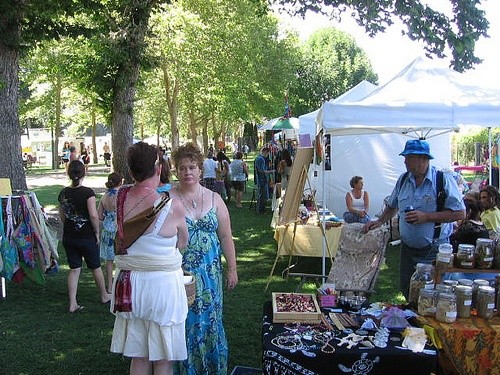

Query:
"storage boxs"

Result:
[[272, 293, 322, 324]]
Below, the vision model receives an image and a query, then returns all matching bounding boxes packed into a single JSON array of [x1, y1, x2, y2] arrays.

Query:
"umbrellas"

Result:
[[259, 115, 302, 131]]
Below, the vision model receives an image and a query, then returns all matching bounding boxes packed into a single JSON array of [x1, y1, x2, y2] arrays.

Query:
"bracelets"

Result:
[[226, 266, 237, 272]]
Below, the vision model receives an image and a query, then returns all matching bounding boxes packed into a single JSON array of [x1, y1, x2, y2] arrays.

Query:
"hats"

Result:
[[399, 140, 434, 160]]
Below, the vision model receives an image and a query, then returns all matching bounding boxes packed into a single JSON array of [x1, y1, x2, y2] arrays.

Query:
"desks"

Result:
[[262, 300, 439, 375], [417, 314, 500, 375], [265, 198, 343, 291]]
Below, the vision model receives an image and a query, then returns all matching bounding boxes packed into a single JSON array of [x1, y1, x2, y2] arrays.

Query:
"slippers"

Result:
[[74, 306, 81, 313]]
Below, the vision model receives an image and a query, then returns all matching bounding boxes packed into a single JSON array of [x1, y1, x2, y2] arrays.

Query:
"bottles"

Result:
[[456, 243, 475, 269], [295, 204, 309, 226], [436, 243, 454, 268], [475, 228, 500, 268], [409, 263, 500, 323]]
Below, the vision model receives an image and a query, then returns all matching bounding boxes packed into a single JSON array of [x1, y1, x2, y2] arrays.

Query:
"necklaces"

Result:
[[130, 181, 154, 192], [179, 185, 201, 209], [176, 183, 204, 221]]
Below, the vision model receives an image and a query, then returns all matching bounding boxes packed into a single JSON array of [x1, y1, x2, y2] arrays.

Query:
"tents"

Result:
[[288, 56, 500, 256]]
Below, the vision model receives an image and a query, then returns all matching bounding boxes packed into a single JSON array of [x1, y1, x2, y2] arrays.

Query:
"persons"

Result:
[[164, 141, 240, 375], [21, 139, 113, 174], [448, 160, 500, 253], [360, 138, 467, 304], [56, 159, 118, 314], [202, 139, 297, 209], [98, 172, 124, 294], [342, 174, 375, 225], [114, 140, 192, 375], [151, 143, 172, 178]]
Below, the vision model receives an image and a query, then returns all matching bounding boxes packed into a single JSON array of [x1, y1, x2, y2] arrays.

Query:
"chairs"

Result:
[[288, 223, 391, 301]]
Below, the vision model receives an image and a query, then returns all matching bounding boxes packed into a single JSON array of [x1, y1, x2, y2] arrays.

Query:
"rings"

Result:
[[232, 280, 235, 283]]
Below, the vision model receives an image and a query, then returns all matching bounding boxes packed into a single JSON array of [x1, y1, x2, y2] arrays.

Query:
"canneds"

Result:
[[404, 205, 414, 210]]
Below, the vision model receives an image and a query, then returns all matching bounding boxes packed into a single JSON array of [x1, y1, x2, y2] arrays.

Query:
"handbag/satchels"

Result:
[[0, 193, 59, 280]]
